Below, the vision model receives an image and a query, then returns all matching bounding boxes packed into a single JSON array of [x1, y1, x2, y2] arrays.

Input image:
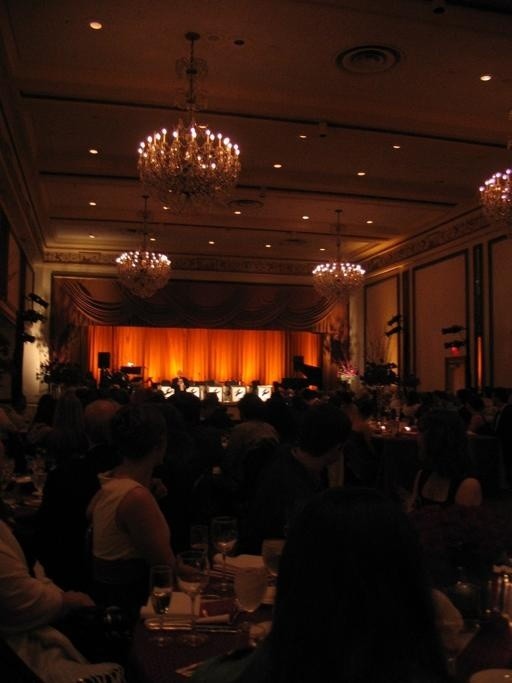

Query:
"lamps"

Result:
[[311, 210, 367, 299], [474, 137, 512, 228], [114, 195, 173, 298], [137, 31, 241, 216]]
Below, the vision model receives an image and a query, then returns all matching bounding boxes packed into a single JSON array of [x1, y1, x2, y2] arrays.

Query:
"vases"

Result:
[[419, 544, 466, 586]]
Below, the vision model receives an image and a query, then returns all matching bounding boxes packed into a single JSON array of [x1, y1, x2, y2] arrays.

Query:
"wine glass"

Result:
[[175, 549, 209, 648], [149, 564, 173, 646], [189, 523, 209, 572], [227, 565, 270, 660], [209, 514, 240, 593]]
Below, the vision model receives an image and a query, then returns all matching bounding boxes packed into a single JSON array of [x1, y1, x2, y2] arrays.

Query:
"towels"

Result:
[[144, 592, 193, 618], [209, 554, 265, 583]]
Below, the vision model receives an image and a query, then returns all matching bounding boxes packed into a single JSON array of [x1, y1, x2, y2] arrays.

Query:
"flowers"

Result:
[[406, 498, 511, 567]]
